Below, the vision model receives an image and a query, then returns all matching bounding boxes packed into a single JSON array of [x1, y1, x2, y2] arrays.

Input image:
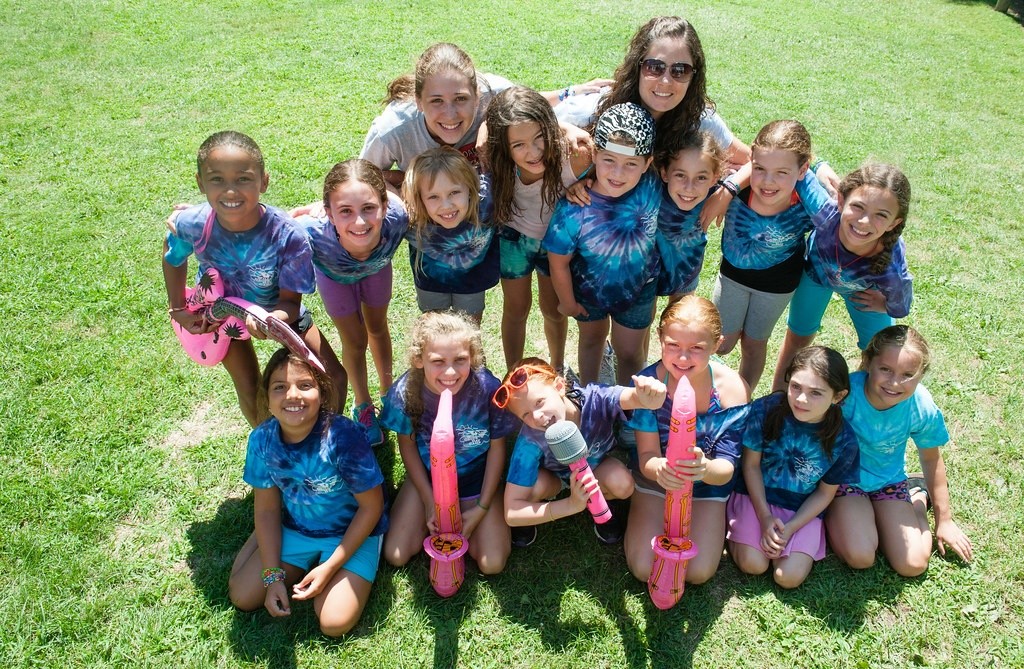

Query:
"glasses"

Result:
[[492, 364, 555, 408], [637, 59, 697, 83]]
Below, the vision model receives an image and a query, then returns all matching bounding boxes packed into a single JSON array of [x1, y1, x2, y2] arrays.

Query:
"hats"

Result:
[[594, 102, 656, 156]]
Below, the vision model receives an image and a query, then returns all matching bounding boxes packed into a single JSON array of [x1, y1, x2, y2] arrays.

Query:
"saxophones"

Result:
[[423, 388, 468, 596], [648, 375, 698, 610]]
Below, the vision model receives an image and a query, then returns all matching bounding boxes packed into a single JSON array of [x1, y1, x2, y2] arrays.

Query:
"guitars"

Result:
[[168, 269, 328, 375]]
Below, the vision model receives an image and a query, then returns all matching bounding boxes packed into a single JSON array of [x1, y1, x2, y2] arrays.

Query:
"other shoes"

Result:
[[906, 471, 949, 496]]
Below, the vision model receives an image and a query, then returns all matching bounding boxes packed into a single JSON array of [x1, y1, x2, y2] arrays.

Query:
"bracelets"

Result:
[[262, 568, 286, 588], [726, 177, 740, 194], [721, 182, 736, 199], [477, 502, 489, 511], [169, 303, 187, 313], [559, 86, 577, 101], [548, 502, 554, 522]]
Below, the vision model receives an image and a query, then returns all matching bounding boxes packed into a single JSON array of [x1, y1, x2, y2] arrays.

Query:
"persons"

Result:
[[165, 15, 841, 446], [162, 130, 348, 430], [228, 348, 386, 636], [726, 346, 860, 589], [825, 325, 973, 577], [621, 295, 751, 585], [380, 312, 516, 575], [700, 160, 913, 393], [492, 357, 666, 547]]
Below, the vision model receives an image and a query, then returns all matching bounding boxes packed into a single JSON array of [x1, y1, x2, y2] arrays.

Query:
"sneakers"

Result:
[[379, 391, 388, 405], [350, 397, 383, 446], [594, 498, 628, 543], [510, 525, 537, 545], [599, 347, 617, 386]]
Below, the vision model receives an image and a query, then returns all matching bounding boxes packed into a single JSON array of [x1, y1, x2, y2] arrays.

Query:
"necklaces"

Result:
[[835, 224, 867, 280]]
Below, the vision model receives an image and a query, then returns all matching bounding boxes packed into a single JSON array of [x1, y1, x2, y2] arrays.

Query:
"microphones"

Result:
[[545, 420, 613, 524]]
